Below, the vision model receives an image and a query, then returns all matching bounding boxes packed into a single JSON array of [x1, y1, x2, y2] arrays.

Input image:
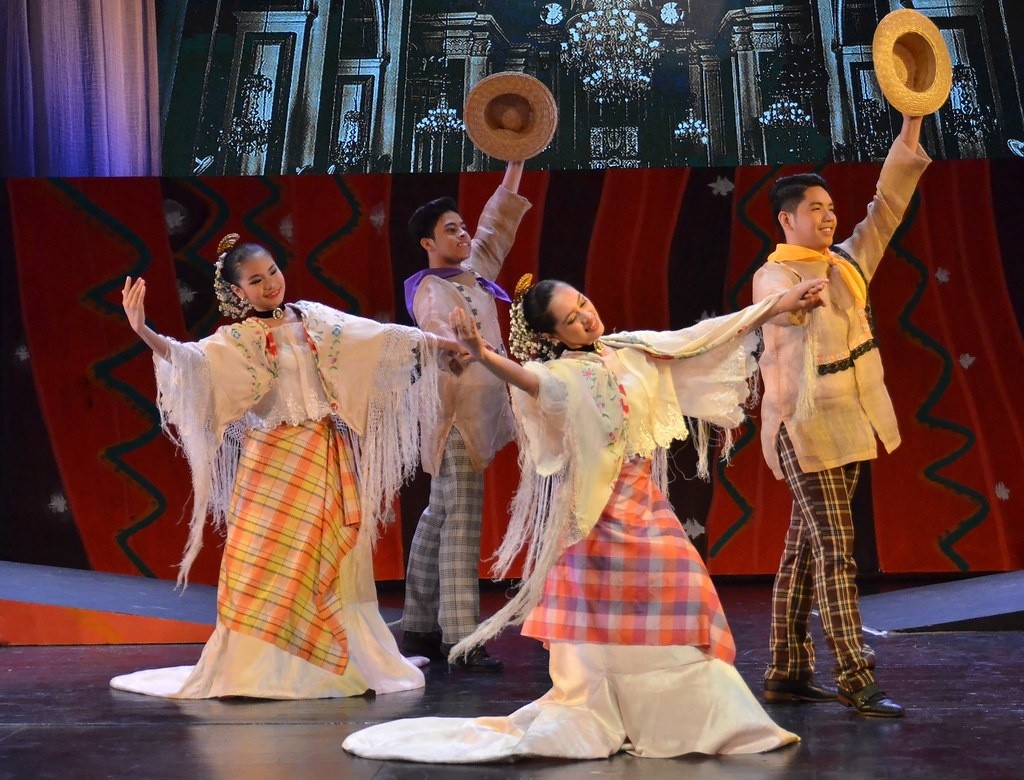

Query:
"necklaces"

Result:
[[565, 337, 605, 355], [253, 301, 286, 321]]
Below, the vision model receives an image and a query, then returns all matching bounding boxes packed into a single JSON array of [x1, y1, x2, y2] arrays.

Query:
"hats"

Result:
[[873, 9, 952, 116], [463, 71, 558, 162]]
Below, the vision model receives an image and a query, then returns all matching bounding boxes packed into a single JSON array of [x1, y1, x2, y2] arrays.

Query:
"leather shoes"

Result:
[[403, 631, 442, 652], [837, 682, 905, 716], [764, 678, 838, 701], [440, 643, 503, 670]]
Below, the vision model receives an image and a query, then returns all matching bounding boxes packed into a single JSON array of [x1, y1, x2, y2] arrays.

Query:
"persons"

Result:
[[749, 112, 923, 716], [396, 158, 527, 672], [118, 242, 499, 699], [447, 276, 833, 758]]
